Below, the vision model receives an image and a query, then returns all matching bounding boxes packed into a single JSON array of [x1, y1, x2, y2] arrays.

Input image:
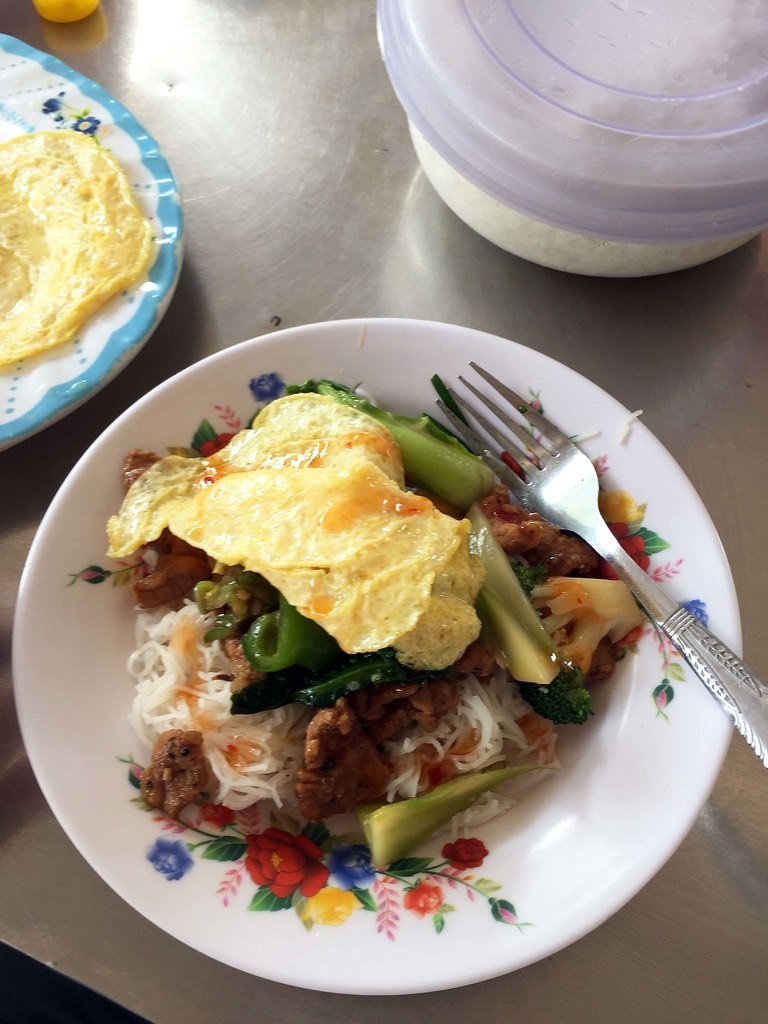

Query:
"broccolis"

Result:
[[520, 663, 596, 723]]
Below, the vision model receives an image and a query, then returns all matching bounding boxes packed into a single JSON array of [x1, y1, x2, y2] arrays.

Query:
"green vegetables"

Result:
[[235, 378, 561, 712]]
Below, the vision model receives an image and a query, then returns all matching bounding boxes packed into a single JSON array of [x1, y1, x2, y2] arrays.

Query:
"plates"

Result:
[[0, 32, 181, 457], [15, 320, 744, 994]]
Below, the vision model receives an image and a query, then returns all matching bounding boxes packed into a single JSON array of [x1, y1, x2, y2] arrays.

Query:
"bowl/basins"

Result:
[[374, 0, 768, 276]]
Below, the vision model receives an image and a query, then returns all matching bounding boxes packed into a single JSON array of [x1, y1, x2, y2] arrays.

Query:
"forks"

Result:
[[435, 361, 768, 774]]
